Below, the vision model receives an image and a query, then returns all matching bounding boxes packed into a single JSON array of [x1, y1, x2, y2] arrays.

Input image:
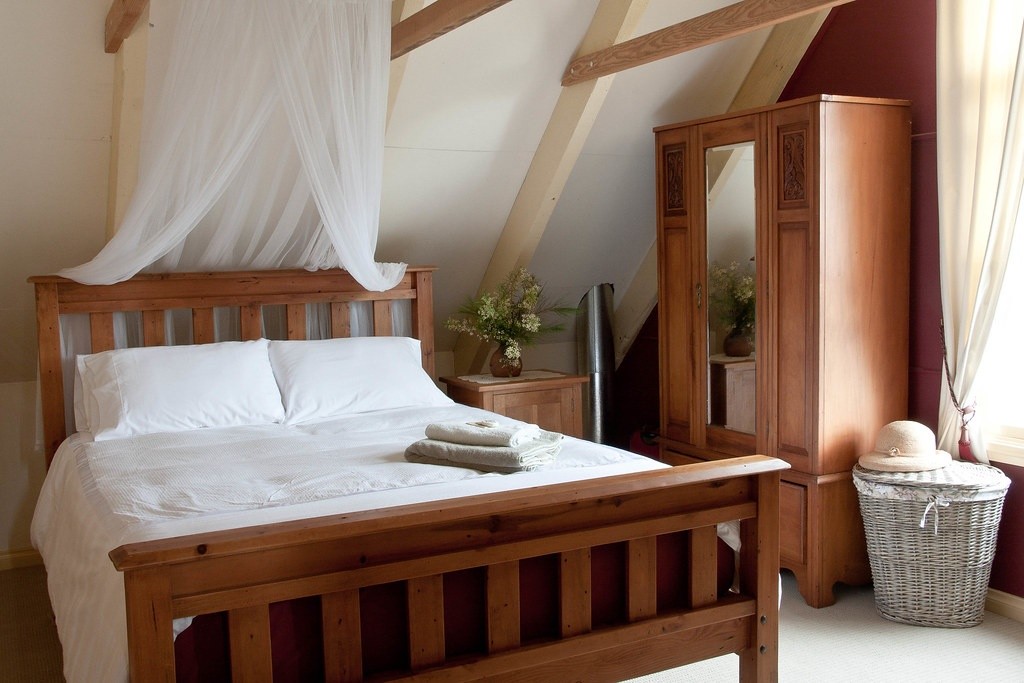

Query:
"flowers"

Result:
[[709, 261, 758, 334], [443, 267, 584, 370]]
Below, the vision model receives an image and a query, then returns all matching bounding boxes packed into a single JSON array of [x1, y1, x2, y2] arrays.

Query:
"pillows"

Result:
[[70, 334, 286, 441], [265, 335, 455, 424]]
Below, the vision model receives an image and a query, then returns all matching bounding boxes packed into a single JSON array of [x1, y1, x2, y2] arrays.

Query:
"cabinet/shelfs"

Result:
[[653, 93, 915, 610]]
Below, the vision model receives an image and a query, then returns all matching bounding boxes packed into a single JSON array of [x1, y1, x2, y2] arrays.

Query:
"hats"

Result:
[[859, 420, 952, 471]]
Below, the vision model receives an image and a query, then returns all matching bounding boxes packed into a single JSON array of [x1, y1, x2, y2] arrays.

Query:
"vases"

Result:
[[721, 325, 754, 358], [490, 344, 523, 377]]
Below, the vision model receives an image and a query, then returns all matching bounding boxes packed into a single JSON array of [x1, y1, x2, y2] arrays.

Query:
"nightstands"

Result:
[[439, 362, 589, 440], [711, 351, 756, 433]]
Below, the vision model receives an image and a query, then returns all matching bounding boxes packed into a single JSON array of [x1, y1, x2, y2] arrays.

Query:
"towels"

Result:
[[403, 429, 566, 474], [424, 418, 542, 448]]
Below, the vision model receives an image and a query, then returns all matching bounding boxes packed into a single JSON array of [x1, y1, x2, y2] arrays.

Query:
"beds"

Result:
[[23, 260, 794, 683]]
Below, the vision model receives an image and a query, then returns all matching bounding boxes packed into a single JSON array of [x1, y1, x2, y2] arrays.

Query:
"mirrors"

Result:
[[706, 138, 757, 433]]
